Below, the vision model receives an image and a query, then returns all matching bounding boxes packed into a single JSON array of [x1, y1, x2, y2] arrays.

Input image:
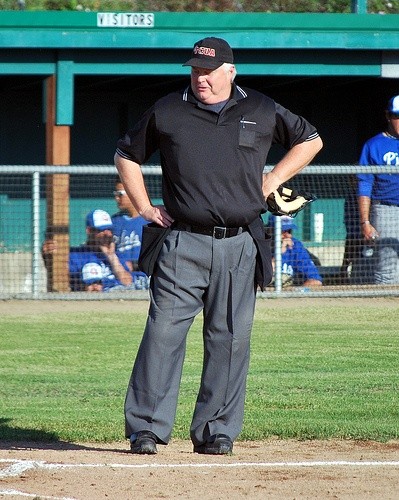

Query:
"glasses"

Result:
[[113, 190, 126, 197]]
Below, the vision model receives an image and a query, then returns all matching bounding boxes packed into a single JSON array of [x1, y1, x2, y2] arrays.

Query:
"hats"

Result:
[[386, 95, 399, 115], [81, 259, 106, 285], [85, 208, 114, 231], [267, 214, 299, 232], [182, 37, 234, 70]]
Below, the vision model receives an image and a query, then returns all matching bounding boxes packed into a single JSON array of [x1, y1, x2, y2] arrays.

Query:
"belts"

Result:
[[370, 200, 399, 208], [170, 223, 247, 240]]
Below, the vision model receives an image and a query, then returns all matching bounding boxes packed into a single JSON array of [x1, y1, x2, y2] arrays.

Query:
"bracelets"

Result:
[[361, 220, 370, 224]]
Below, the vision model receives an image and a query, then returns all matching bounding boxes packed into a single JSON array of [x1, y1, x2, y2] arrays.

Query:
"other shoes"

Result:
[[129, 431, 158, 455], [192, 434, 234, 455]]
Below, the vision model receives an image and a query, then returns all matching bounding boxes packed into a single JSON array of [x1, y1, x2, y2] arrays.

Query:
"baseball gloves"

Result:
[[266, 185, 318, 216]]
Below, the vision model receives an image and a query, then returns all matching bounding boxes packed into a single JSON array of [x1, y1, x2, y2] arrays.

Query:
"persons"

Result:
[[41, 96, 399, 291], [115, 38, 324, 455]]
